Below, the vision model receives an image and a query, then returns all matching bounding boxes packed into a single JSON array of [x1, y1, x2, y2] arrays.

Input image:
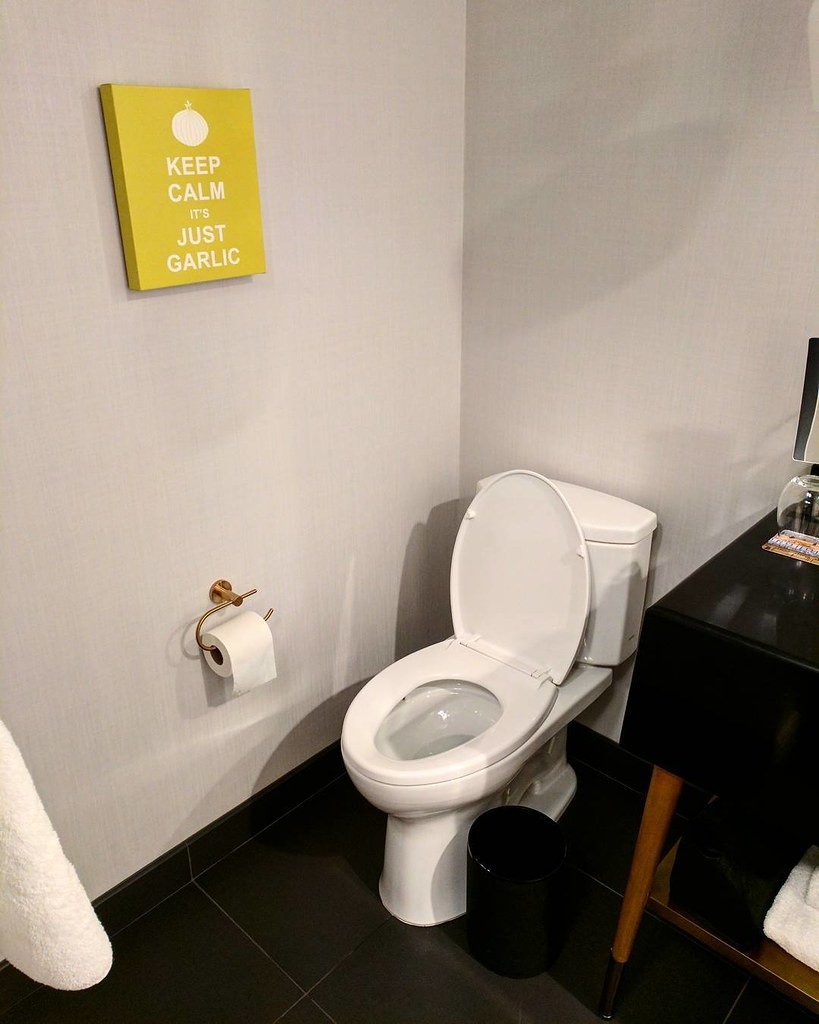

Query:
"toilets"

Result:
[[339, 470, 660, 927]]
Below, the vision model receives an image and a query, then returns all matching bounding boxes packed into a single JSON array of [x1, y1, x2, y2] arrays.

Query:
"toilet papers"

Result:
[[200, 610, 278, 696]]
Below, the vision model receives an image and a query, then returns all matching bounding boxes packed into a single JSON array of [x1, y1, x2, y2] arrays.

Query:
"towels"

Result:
[[0, 721, 113, 991], [762, 845, 819, 974]]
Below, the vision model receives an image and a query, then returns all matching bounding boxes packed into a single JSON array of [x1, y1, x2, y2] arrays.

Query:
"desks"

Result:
[[598, 503, 819, 1024]]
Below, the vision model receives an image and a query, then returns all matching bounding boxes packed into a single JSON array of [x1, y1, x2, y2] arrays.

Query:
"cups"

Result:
[[777, 475, 819, 544]]
